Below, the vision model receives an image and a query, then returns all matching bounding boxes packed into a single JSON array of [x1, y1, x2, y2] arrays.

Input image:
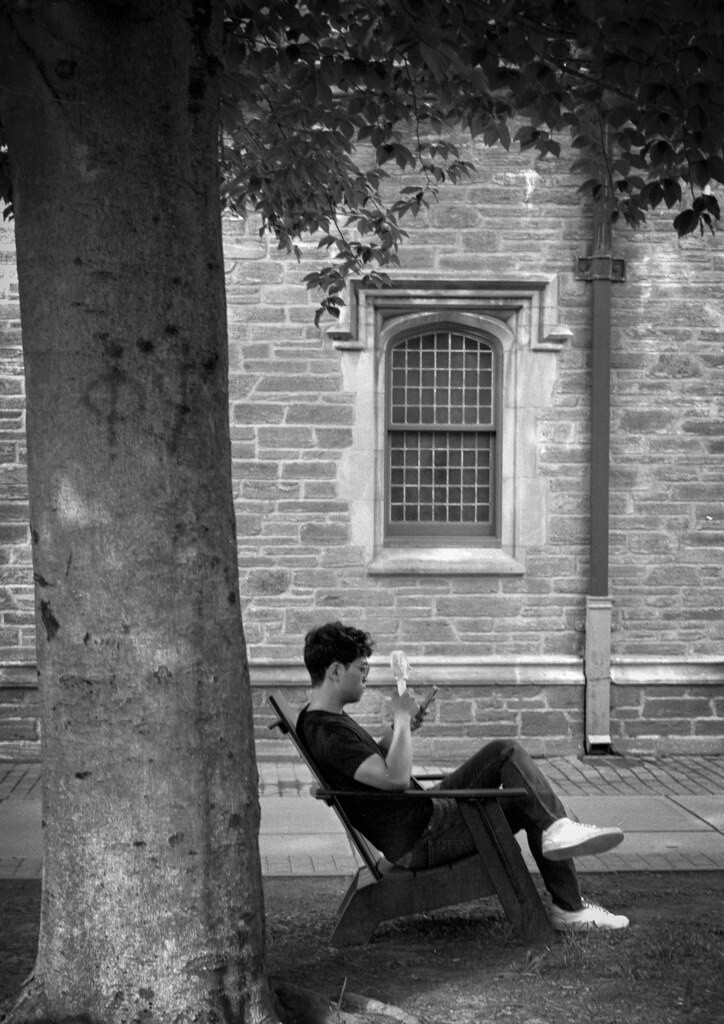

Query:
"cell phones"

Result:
[[414, 685, 439, 727]]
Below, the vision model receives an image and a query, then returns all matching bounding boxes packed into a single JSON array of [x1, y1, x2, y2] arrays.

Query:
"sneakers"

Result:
[[551, 896, 629, 932], [542, 809, 624, 861]]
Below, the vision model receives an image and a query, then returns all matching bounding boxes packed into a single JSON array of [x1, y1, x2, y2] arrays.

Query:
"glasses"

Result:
[[348, 663, 370, 676]]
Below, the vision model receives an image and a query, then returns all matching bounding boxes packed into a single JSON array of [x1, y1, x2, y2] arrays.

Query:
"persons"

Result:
[[295, 622, 629, 928]]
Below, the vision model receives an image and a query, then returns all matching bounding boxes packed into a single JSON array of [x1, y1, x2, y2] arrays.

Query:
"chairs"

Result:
[[267, 686, 560, 952]]
